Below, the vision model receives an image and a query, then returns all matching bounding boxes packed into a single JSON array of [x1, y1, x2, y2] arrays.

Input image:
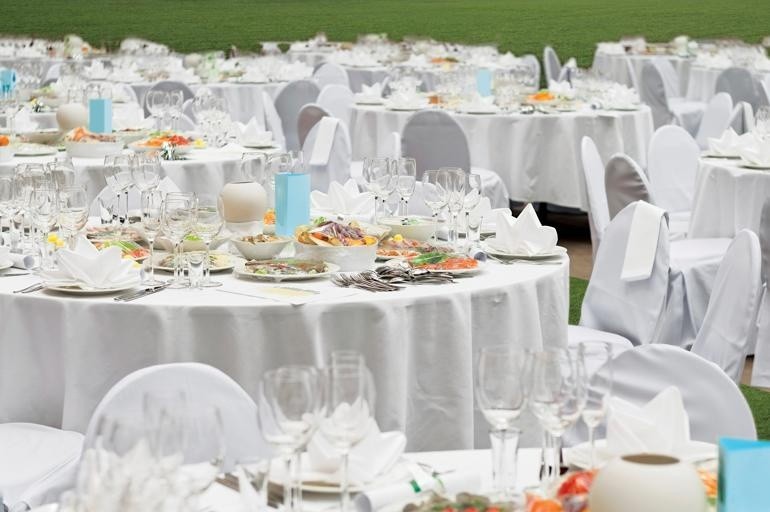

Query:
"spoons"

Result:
[[376, 265, 453, 285]]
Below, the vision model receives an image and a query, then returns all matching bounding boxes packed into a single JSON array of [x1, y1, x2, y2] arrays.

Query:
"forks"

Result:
[[487, 251, 562, 266], [332, 272, 405, 293]]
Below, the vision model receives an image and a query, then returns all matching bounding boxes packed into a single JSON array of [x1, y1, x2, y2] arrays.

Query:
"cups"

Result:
[[59, 65, 114, 106], [494, 64, 537, 92], [98, 196, 114, 225], [240, 150, 304, 192]]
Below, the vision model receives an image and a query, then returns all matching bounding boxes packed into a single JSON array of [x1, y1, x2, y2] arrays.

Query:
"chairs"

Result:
[[1, 33, 770, 512]]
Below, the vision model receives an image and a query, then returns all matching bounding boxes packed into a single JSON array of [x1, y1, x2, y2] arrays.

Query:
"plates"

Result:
[[143, 251, 238, 271], [128, 144, 163, 154], [483, 237, 568, 258], [707, 154, 741, 159], [244, 141, 274, 148], [744, 163, 770, 170], [42, 278, 136, 295], [233, 79, 266, 85], [349, 95, 638, 115], [12, 147, 59, 157], [87, 236, 141, 244], [232, 257, 341, 280], [458, 232, 495, 243], [376, 247, 482, 274]]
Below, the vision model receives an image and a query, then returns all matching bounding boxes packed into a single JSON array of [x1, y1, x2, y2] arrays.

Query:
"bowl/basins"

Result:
[[293, 237, 379, 272], [63, 137, 126, 160], [19, 131, 63, 144], [230, 233, 291, 262]]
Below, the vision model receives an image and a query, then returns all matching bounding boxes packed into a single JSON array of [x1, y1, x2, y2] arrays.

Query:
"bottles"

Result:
[[57, 105, 90, 132], [263, 206, 275, 235], [217, 180, 268, 222], [588, 448, 707, 512]]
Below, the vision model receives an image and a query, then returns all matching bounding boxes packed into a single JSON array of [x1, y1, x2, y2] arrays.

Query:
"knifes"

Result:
[[113, 284, 168, 302]]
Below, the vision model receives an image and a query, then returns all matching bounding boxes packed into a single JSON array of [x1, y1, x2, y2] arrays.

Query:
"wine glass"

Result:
[[0, 156, 90, 272], [103, 151, 223, 288], [146, 91, 236, 150], [0, 60, 40, 147], [362, 153, 483, 247], [59, 342, 616, 512]]
